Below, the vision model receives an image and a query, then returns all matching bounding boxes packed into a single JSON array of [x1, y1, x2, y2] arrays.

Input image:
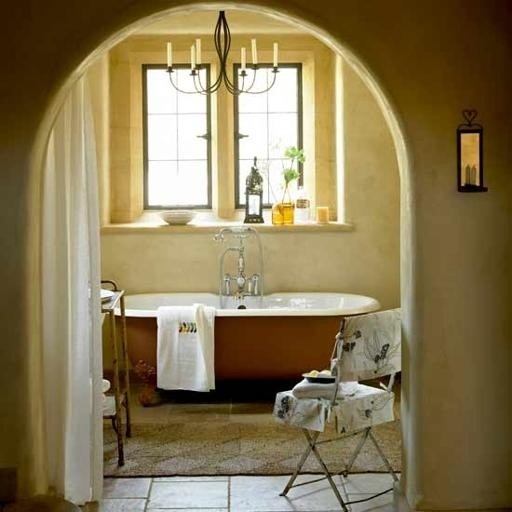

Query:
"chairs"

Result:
[[273, 307, 402, 512]]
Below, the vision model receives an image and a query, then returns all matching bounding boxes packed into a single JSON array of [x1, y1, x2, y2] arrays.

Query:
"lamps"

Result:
[[167, 11, 280, 95]]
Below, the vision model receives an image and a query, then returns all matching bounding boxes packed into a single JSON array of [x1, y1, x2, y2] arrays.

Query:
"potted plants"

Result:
[[271, 145, 306, 225]]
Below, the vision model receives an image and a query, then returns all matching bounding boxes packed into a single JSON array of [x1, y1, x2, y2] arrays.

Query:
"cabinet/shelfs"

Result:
[[100, 279, 133, 467]]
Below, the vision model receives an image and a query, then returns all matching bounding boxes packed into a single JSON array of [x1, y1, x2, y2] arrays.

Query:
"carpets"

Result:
[[103, 422, 402, 477]]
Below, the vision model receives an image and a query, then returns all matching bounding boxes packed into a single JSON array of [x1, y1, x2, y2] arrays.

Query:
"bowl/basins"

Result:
[[158, 209, 199, 225]]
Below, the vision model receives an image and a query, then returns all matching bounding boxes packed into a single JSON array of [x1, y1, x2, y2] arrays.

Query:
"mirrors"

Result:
[[456, 109, 488, 193]]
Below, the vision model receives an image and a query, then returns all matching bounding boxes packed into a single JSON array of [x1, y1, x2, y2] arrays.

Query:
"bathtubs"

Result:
[[114, 289, 381, 378]]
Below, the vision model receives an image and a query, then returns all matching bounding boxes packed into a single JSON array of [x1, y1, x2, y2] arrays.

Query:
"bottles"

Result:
[[295, 185, 310, 224]]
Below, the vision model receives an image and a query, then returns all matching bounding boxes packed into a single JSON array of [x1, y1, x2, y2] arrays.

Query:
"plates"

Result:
[[302, 372, 336, 382], [88, 288, 114, 304]]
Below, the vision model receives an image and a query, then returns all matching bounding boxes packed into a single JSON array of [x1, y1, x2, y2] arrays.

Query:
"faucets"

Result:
[[222, 254, 260, 300]]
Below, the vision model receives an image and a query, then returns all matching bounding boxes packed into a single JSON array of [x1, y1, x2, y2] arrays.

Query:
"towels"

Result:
[[101, 379, 117, 416], [293, 378, 359, 398], [156, 304, 215, 393]]
